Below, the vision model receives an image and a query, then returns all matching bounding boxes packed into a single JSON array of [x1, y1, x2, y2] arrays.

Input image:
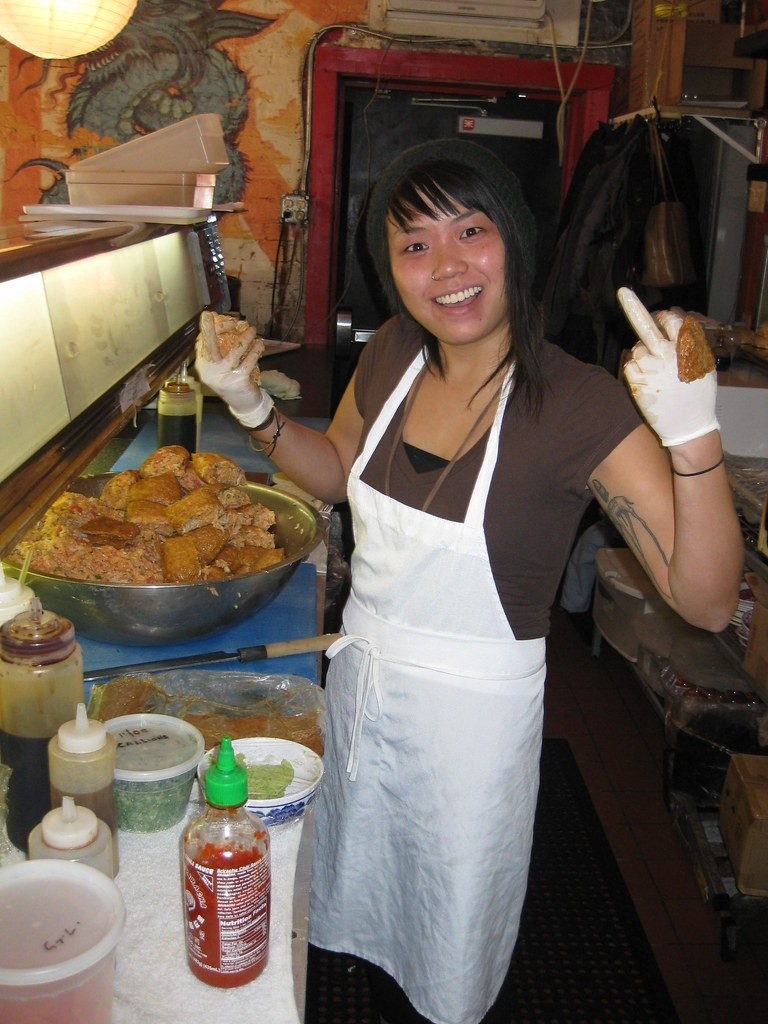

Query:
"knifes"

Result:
[[82, 633, 343, 682]]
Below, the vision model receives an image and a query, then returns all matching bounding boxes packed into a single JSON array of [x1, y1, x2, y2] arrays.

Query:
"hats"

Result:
[[367, 138, 536, 303]]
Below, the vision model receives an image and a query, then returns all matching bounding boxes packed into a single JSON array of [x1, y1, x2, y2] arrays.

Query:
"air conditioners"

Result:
[[385, 0, 546, 30]]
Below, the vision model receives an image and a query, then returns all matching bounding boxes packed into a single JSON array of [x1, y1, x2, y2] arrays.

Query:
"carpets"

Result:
[[305, 738, 680, 1024]]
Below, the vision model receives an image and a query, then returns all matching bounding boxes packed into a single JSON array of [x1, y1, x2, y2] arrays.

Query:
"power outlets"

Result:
[[282, 195, 309, 223]]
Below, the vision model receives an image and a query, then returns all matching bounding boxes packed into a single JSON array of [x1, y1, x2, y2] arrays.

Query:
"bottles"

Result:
[[182, 737, 271, 988], [164, 363, 203, 453], [0, 597, 83, 853], [0, 565, 43, 627], [158, 376, 197, 461], [712, 324, 731, 371], [28, 796, 115, 880], [48, 702, 121, 877]]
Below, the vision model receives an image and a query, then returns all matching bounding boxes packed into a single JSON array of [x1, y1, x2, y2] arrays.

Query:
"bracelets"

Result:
[[244, 405, 286, 458], [673, 454, 725, 477]]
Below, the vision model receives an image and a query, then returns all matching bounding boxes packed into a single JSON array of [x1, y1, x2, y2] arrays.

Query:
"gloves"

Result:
[[194, 310, 275, 430], [616, 287, 721, 448]]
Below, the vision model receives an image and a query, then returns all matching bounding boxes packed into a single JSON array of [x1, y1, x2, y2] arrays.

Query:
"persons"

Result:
[[195, 139, 745, 1024]]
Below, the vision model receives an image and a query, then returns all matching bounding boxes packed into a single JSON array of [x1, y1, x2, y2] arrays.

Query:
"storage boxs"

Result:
[[719, 753, 768, 896], [65, 170, 217, 208], [591, 545, 676, 662], [70, 113, 230, 173]]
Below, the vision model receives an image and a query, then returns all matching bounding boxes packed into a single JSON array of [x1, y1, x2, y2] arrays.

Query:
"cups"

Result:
[[0, 860, 127, 1024]]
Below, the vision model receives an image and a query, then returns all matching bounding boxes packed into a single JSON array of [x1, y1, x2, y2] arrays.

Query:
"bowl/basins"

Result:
[[0, 472, 325, 647], [198, 737, 324, 834], [101, 714, 206, 834]]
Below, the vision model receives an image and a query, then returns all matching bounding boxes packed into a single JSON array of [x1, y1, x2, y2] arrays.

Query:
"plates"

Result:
[[729, 583, 757, 649]]
